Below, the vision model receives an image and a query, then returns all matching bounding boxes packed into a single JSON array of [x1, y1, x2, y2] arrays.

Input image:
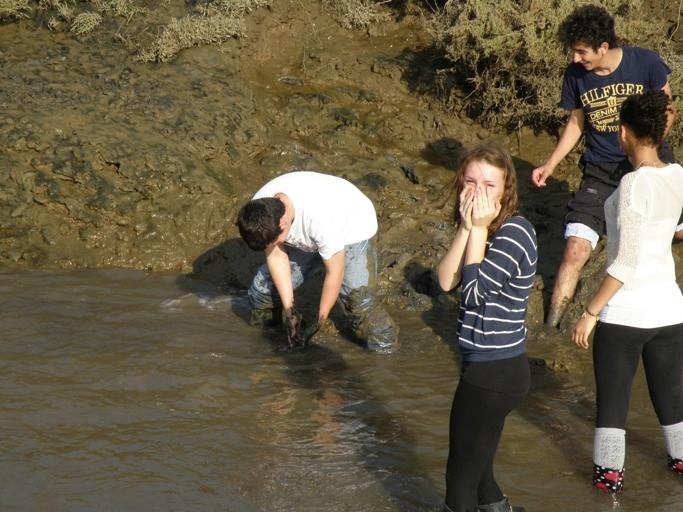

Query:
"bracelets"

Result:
[[584, 305, 597, 319]]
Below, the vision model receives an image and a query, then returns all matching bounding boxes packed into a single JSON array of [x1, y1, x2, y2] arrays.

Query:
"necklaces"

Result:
[[633, 159, 660, 171]]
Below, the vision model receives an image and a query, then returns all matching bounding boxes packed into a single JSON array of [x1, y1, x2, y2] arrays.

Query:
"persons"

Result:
[[569, 92, 682, 497], [433, 140, 540, 511], [529, 4, 677, 329], [234, 171, 399, 358]]
